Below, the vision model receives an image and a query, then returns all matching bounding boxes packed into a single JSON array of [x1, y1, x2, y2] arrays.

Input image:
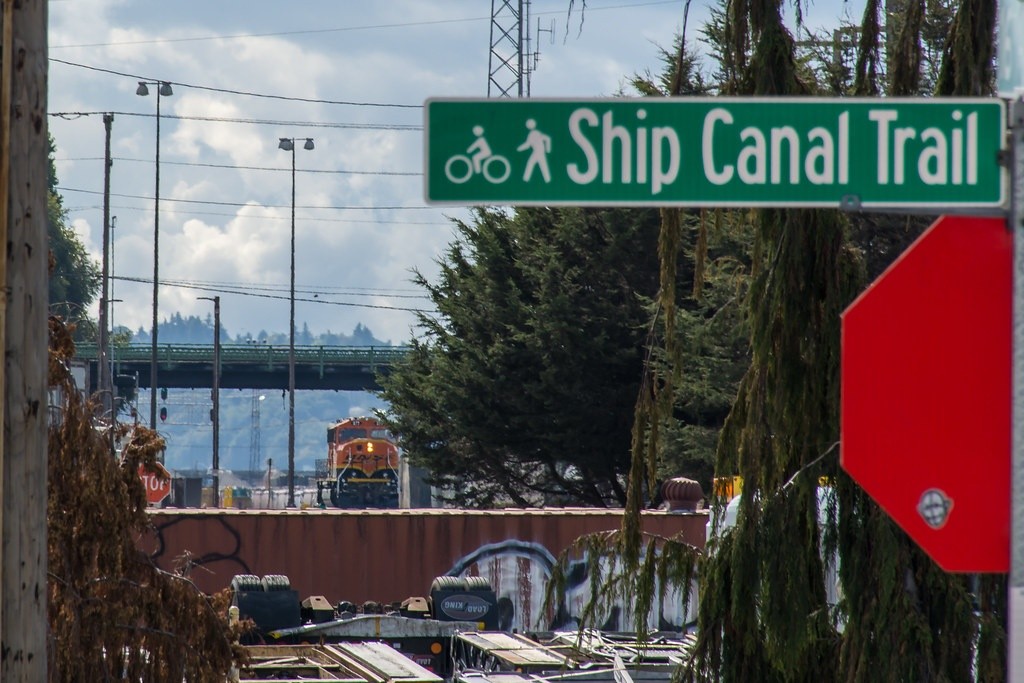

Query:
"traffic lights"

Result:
[[159, 407, 168, 423]]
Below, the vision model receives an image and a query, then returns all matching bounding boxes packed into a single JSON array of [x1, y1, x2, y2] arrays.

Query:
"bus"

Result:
[[325, 415, 400, 510]]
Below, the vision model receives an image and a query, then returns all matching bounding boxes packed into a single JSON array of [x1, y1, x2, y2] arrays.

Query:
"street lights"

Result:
[[271, 135, 317, 509], [136, 80, 173, 453], [195, 295, 220, 510]]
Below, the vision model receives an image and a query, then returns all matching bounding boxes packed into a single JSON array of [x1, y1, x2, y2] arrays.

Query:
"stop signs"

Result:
[[137, 461, 172, 505]]
